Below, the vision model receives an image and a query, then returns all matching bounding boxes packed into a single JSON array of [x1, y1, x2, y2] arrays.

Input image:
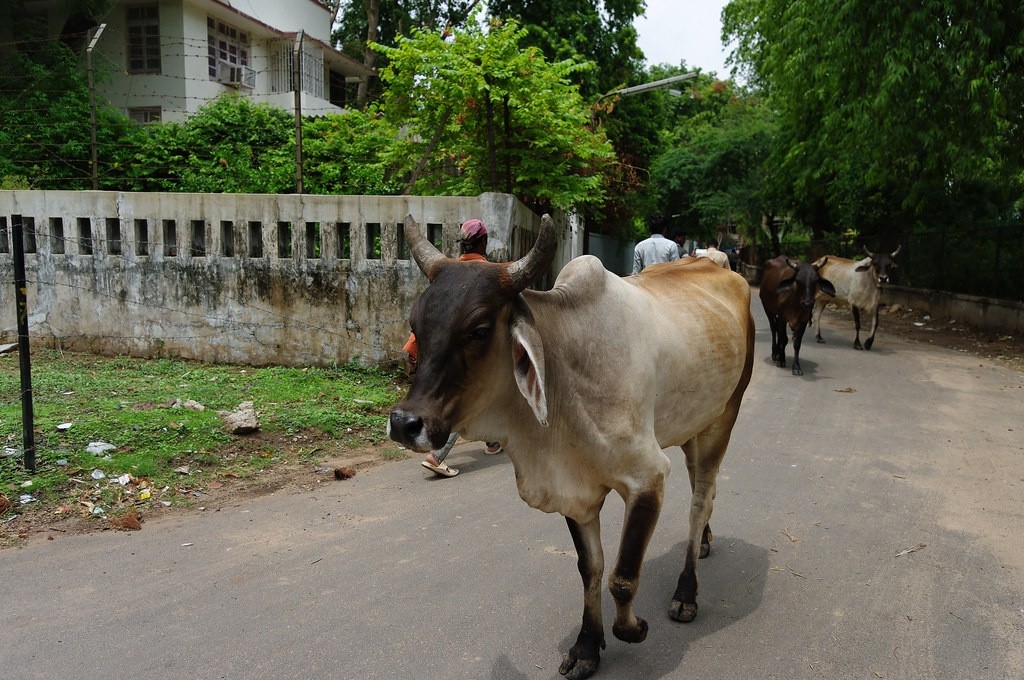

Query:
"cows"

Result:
[[384, 213, 757, 680], [759, 245, 902, 376]]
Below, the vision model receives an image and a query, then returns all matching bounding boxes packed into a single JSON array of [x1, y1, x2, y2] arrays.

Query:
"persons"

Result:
[[728, 244, 745, 272], [670, 228, 687, 259], [696, 239, 731, 270], [632, 214, 680, 273], [403, 219, 502, 476]]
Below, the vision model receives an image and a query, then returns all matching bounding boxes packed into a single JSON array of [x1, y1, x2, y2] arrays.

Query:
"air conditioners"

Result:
[[221, 66, 257, 88]]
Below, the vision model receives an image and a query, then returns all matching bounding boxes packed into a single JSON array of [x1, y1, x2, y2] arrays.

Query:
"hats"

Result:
[[455, 219, 487, 245]]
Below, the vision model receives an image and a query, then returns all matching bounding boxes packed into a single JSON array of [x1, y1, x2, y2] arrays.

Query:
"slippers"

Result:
[[422, 460, 459, 477], [484, 445, 503, 455]]
